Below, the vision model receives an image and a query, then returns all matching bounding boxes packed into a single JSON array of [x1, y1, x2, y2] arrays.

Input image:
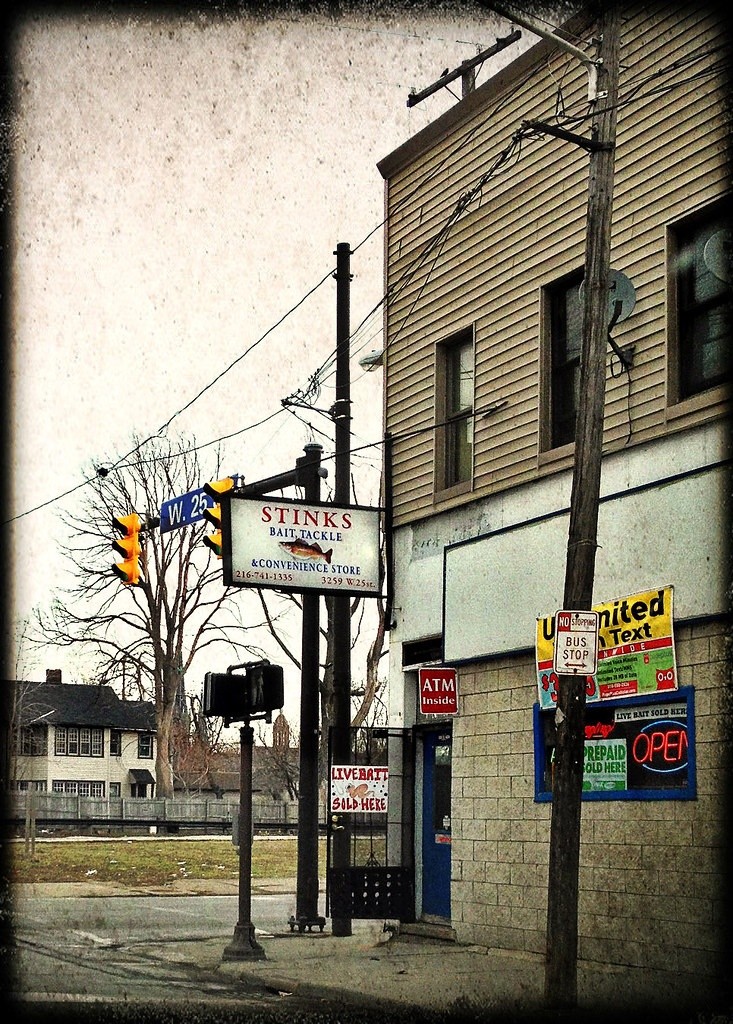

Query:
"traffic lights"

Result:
[[110, 514, 140, 585], [200, 474, 234, 560]]
